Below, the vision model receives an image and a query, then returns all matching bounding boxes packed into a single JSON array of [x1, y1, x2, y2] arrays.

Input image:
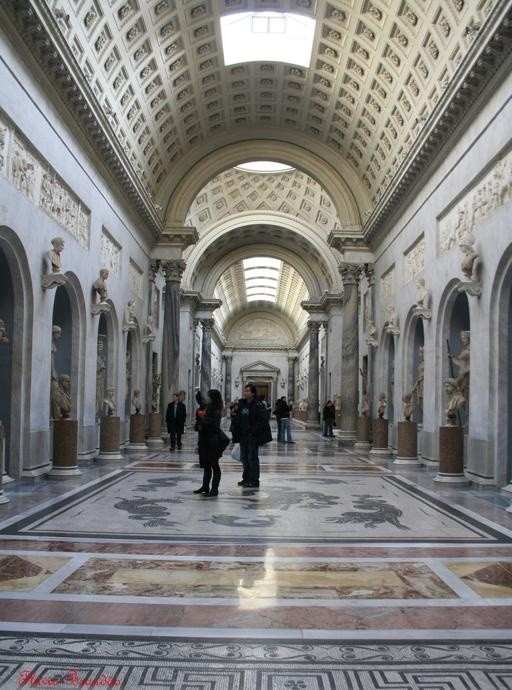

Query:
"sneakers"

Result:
[[192, 487, 218, 497], [237, 480, 260, 488]]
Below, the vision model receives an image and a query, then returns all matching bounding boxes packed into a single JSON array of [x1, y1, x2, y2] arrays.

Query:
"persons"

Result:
[[45, 234, 65, 277], [376, 391, 390, 418], [384, 304, 398, 329], [278, 395, 296, 444], [413, 345, 423, 424], [366, 320, 376, 342], [97, 333, 108, 414], [194, 390, 229, 498], [195, 391, 208, 430], [460, 232, 477, 278], [276, 398, 281, 443], [401, 389, 416, 418], [231, 382, 273, 489], [103, 383, 118, 417], [447, 328, 470, 379], [323, 400, 337, 438], [53, 372, 74, 419], [147, 315, 154, 335], [167, 393, 185, 454], [52, 324, 61, 372], [359, 395, 369, 416], [413, 277, 432, 310], [150, 392, 160, 412], [402, 240, 425, 275], [131, 389, 142, 415], [1, 123, 89, 243], [441, 377, 465, 419], [100, 234, 121, 273], [440, 151, 512, 247], [94, 267, 110, 300], [126, 299, 137, 326]]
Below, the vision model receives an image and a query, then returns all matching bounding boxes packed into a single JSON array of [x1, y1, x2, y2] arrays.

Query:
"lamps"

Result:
[[235, 377, 239, 386], [281, 378, 285, 387]]
